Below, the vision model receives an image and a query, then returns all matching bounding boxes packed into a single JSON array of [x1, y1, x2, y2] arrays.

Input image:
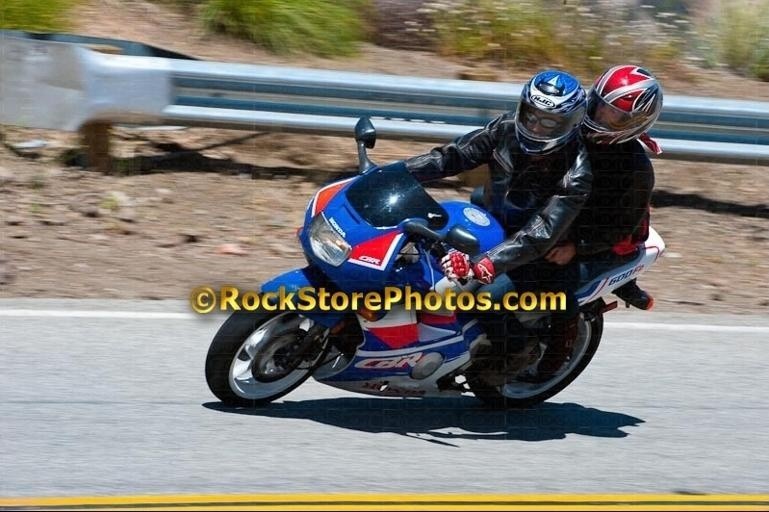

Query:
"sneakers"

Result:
[[478, 334, 541, 388], [554, 326, 579, 359]]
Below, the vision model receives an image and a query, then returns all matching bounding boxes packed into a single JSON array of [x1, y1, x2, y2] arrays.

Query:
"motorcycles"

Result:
[[205, 115, 667, 413]]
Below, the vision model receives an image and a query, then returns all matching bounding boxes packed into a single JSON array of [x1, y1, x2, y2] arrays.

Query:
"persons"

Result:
[[526, 62, 666, 376], [400, 68, 592, 389]]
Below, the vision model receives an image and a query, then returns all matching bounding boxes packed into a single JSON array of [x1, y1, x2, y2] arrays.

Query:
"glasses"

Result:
[[522, 109, 563, 130]]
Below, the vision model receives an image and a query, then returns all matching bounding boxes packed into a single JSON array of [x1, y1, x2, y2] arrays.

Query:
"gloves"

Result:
[[439, 247, 494, 287]]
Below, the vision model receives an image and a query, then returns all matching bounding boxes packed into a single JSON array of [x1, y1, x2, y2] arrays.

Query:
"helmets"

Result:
[[514, 70, 588, 159], [583, 64, 664, 153]]
